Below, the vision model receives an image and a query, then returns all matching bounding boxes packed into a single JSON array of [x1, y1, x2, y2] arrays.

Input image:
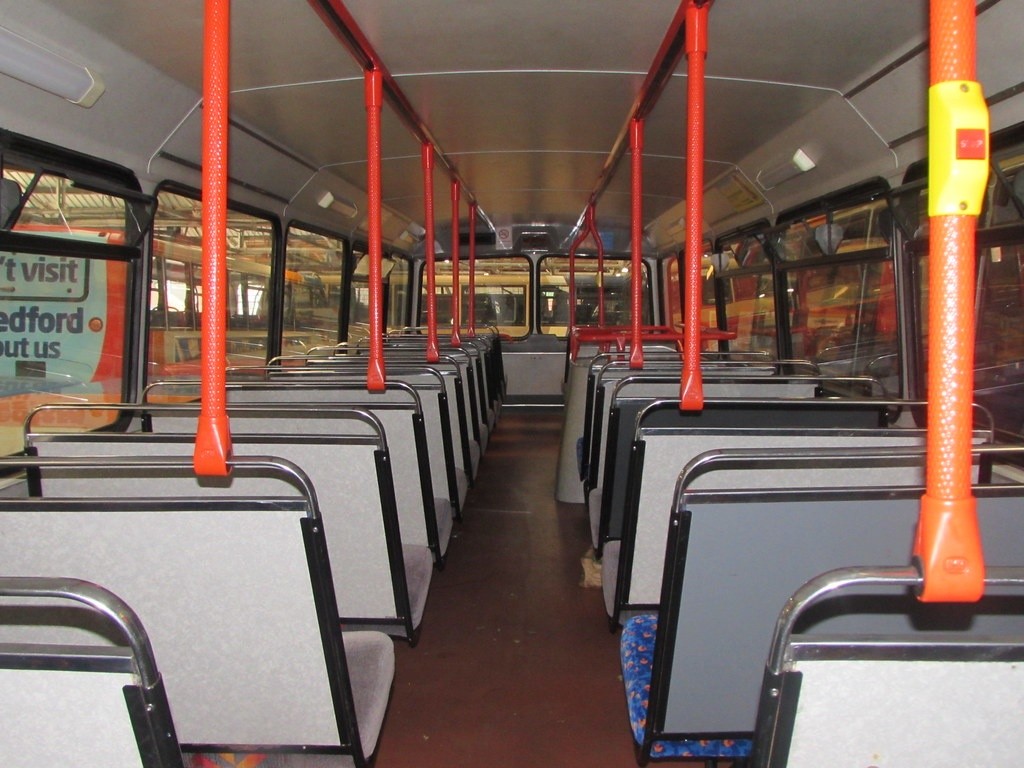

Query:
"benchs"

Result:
[[618, 443, 1024, 768], [748, 565, 1024, 768], [24, 405, 434, 648], [576, 352, 781, 482], [333, 342, 495, 436], [583, 360, 824, 511], [224, 365, 468, 525], [0, 575, 184, 768], [265, 355, 482, 490], [382, 326, 508, 417], [0, 455, 397, 768], [354, 338, 501, 427], [587, 374, 888, 564], [140, 379, 456, 572], [305, 344, 488, 462], [600, 397, 996, 635]]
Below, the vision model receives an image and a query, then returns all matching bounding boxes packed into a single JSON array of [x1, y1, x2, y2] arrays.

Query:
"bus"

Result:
[[0, 222, 368, 459], [255, 270, 649, 339], [671, 157, 1024, 443]]
